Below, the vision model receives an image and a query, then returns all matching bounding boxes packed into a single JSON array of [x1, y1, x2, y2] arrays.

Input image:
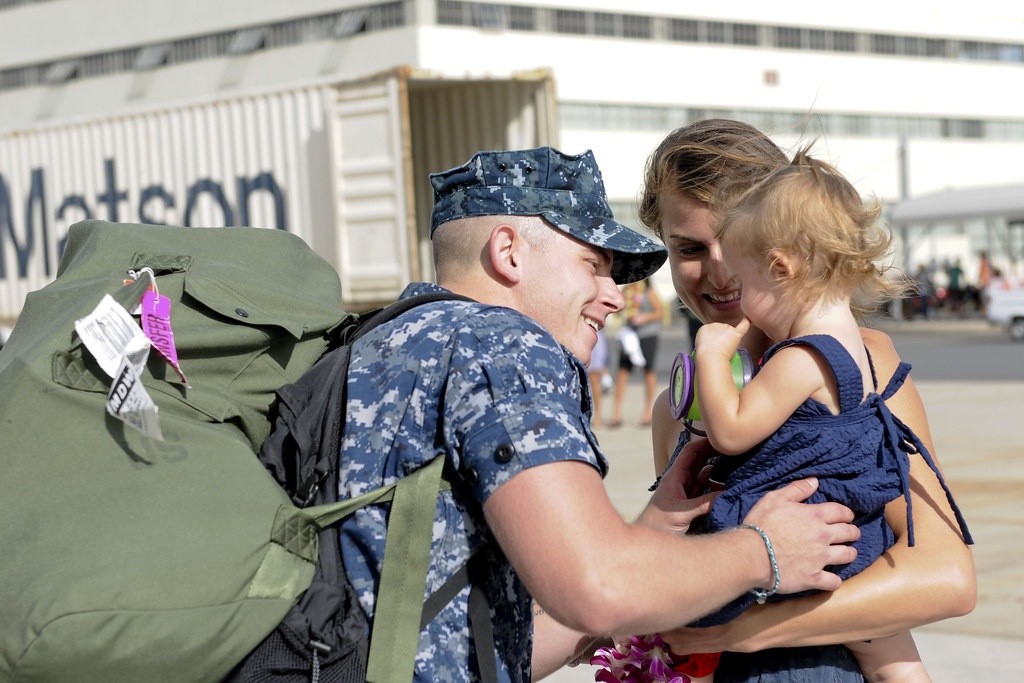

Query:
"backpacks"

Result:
[[0, 219, 459, 683]]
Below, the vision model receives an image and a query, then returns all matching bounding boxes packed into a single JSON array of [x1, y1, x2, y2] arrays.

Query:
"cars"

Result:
[[984, 278, 1024, 344]]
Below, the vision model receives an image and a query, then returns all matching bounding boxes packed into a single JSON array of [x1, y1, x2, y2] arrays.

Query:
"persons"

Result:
[[586, 322, 606, 428], [669, 150, 973, 628], [596, 120, 975, 683], [913, 252, 999, 320], [734, 524, 781, 598], [609, 273, 663, 427], [223, 146, 861, 683]]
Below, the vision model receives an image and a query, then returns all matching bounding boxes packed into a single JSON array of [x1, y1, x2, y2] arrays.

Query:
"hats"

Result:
[[429, 146, 669, 284]]
